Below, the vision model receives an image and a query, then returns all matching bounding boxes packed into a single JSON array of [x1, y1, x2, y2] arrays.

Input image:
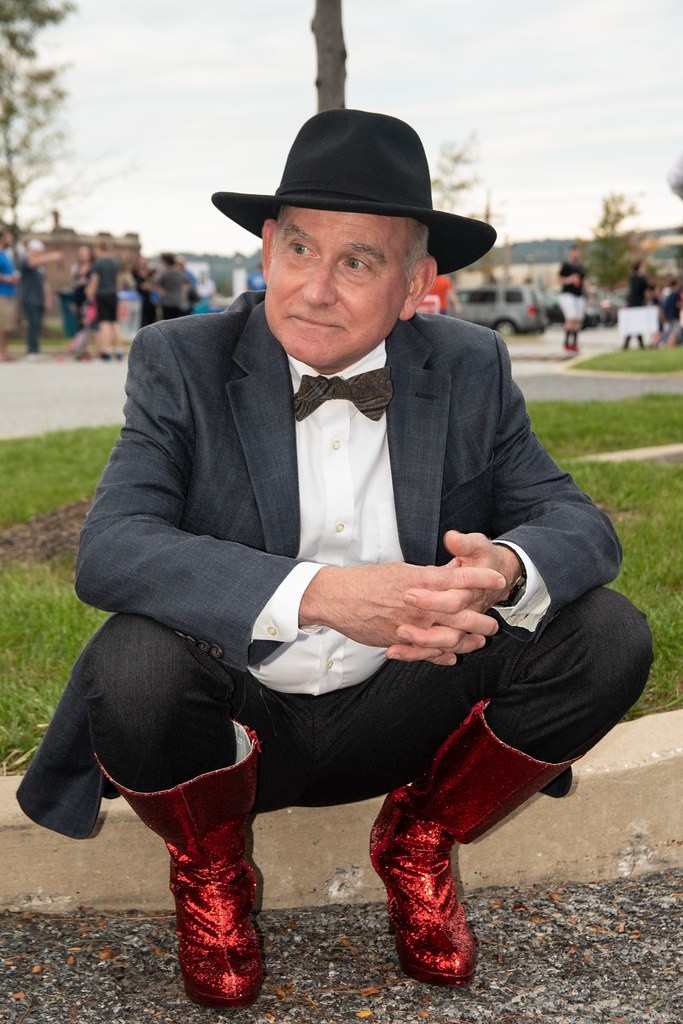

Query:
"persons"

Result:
[[555, 242, 587, 355], [416, 272, 461, 316], [8, 108, 659, 1008], [0, 229, 201, 363], [619, 259, 682, 351], [247, 264, 266, 290]]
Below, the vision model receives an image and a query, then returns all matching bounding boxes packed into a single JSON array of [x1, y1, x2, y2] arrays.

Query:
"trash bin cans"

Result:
[[58, 292, 80, 337]]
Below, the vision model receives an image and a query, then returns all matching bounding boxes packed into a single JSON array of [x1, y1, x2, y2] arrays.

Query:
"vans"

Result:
[[448, 284, 549, 335]]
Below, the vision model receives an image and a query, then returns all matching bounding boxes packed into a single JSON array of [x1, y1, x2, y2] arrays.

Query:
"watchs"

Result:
[[493, 542, 527, 608]]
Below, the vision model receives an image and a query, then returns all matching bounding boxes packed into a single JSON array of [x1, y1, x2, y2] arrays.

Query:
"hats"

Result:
[[28, 240, 44, 250], [211, 109, 497, 275]]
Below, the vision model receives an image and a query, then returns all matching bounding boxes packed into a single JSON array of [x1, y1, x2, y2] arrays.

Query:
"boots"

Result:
[[93, 721, 265, 1008], [369, 700, 586, 987]]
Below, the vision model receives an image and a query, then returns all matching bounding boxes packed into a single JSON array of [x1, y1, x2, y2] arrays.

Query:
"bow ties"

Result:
[[295, 366, 392, 422]]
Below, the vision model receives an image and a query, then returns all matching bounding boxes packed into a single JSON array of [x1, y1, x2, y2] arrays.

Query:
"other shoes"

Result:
[[573, 344, 578, 350], [565, 345, 571, 350]]
